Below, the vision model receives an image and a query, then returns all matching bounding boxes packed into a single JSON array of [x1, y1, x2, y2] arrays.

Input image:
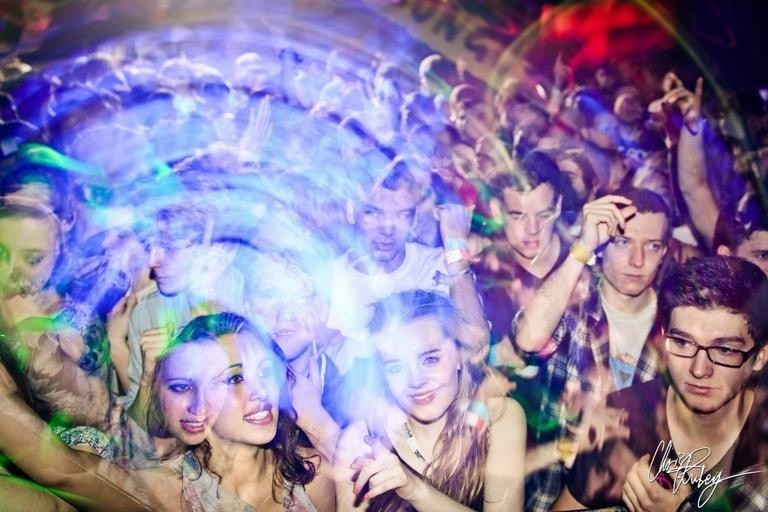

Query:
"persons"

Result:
[[1, 1, 767, 512]]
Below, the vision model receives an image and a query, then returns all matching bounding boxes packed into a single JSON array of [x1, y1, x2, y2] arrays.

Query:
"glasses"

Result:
[[659, 331, 766, 371]]
